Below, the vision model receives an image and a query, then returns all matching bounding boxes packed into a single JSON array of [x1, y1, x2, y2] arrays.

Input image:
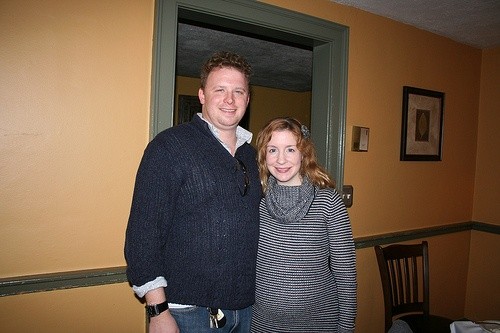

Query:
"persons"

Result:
[[248, 116, 357, 333], [125, 49, 265, 333]]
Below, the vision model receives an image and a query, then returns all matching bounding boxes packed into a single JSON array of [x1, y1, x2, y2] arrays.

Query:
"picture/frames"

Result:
[[400, 85, 447, 164]]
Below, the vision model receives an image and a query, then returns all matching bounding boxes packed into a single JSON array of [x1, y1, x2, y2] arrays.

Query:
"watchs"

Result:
[[145, 300, 169, 318]]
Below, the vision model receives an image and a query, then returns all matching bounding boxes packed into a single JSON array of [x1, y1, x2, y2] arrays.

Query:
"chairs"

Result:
[[374, 239, 459, 333]]
